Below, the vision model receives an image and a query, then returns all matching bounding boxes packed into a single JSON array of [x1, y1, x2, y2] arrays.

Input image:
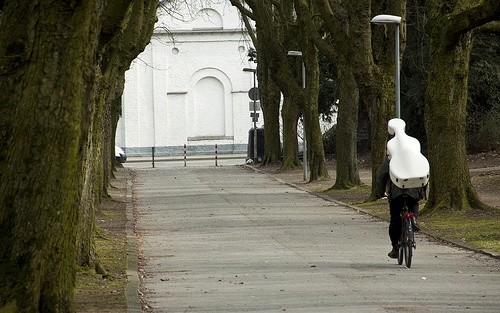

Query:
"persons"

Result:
[[374, 155, 428, 258]]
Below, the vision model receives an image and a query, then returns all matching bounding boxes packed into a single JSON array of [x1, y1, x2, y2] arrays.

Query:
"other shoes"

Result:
[[412, 215, 421, 231], [387, 242, 399, 258]]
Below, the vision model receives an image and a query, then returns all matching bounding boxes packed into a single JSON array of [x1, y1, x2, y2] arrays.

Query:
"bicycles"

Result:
[[383, 191, 421, 268]]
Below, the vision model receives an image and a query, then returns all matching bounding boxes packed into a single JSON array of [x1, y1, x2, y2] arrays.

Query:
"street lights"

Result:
[[287, 50, 308, 181], [371, 14, 402, 118], [242, 68, 258, 164]]
